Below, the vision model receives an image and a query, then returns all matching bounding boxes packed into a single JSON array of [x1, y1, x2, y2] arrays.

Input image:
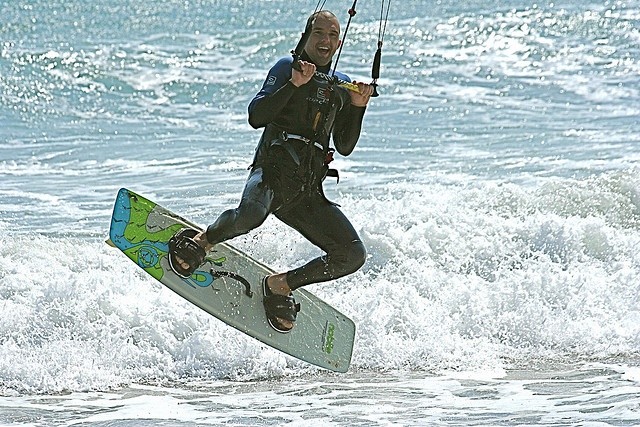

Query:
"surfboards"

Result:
[[105, 187, 356, 373]]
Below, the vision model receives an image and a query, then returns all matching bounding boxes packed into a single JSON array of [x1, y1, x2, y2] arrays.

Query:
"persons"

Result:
[[173, 8, 373, 330]]
